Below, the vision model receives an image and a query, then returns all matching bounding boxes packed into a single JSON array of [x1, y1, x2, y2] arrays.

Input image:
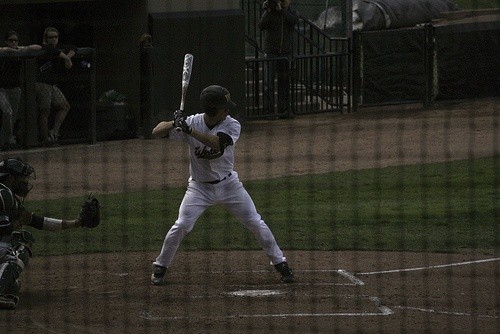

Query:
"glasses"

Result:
[[10, 39, 18, 41], [48, 35, 58, 38]]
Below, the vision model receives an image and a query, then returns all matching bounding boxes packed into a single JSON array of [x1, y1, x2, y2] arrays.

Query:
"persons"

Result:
[[0, 158, 100, 309], [128, 34, 158, 138], [0, 27, 78, 146], [257, 0, 300, 120], [151, 84, 295, 283]]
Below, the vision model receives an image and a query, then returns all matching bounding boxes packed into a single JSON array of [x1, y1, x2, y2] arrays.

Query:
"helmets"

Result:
[[198, 84, 237, 115], [0, 157, 37, 198]]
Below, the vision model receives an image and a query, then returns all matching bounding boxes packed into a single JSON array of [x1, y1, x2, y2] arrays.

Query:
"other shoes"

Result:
[[279, 111, 294, 119], [264, 115, 278, 120], [48, 129, 58, 142], [3, 137, 17, 149]]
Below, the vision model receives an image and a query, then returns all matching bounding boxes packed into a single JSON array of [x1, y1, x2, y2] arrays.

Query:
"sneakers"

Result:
[[274, 262, 296, 283], [151, 264, 167, 287]]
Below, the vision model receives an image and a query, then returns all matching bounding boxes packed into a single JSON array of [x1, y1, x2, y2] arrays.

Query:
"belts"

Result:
[[203, 173, 233, 185]]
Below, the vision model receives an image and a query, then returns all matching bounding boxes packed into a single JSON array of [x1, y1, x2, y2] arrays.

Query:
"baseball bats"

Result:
[[175, 53, 194, 133]]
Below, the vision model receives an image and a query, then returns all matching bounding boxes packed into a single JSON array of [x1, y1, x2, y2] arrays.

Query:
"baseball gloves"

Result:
[[79, 191, 100, 228]]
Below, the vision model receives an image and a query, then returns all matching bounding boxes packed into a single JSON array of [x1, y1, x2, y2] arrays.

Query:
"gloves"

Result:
[[174, 110, 194, 136]]
